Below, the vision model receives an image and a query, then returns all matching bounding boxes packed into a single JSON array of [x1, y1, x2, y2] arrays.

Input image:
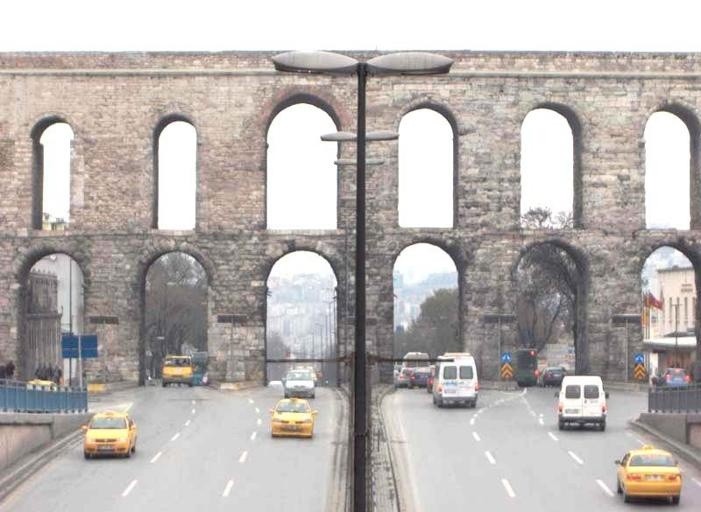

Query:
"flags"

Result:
[[643, 292, 662, 310]]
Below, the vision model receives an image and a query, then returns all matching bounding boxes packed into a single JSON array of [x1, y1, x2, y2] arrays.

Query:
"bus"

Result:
[[513, 347, 538, 388]]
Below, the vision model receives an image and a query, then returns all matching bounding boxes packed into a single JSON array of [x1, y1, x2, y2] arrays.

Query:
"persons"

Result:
[[5, 360, 15, 379]]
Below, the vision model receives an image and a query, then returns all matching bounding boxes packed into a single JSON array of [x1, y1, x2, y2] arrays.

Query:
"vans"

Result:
[[160, 355, 196, 388], [553, 374, 609, 433]]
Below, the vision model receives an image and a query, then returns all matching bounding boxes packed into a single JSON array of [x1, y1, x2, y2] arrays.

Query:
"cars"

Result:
[[538, 366, 568, 389], [280, 369, 315, 399], [24, 378, 57, 393], [79, 409, 138, 459], [612, 445, 684, 506], [654, 366, 693, 388], [266, 396, 319, 440]]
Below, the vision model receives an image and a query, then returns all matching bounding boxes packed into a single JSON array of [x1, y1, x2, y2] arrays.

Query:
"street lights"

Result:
[[610, 313, 641, 382], [317, 129, 401, 206], [299, 294, 337, 391], [270, 51, 454, 512], [670, 302, 683, 369], [217, 314, 250, 385], [481, 313, 515, 380], [86, 314, 120, 385]]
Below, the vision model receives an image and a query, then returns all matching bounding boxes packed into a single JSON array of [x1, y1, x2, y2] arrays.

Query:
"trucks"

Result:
[[393, 346, 481, 409]]
